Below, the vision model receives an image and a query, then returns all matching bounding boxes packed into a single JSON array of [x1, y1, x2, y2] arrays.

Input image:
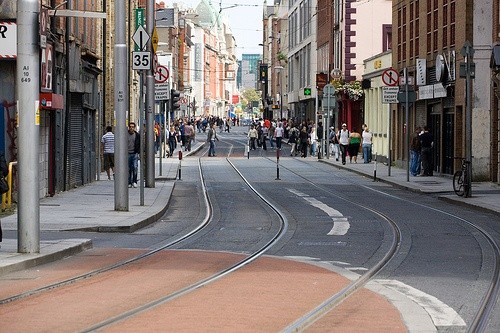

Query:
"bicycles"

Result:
[[448, 157, 472, 198]]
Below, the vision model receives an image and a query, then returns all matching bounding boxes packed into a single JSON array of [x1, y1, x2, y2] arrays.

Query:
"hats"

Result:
[[342, 123, 347, 127]]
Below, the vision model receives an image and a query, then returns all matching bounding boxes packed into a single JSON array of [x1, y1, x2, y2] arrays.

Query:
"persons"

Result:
[[99, 114, 239, 188], [246, 118, 435, 178], [0, 151, 9, 249]]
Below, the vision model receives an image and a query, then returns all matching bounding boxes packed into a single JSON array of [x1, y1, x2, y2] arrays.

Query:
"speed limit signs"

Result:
[[132, 51, 151, 71]]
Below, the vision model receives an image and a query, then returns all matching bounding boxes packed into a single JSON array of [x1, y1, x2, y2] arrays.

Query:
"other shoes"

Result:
[[132, 182, 138, 188], [343, 162, 345, 165], [420, 174, 428, 176], [128, 184, 132, 188]]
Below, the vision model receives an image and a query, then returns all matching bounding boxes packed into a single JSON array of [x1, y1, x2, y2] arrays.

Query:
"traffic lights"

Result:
[[169, 88, 180, 113]]
